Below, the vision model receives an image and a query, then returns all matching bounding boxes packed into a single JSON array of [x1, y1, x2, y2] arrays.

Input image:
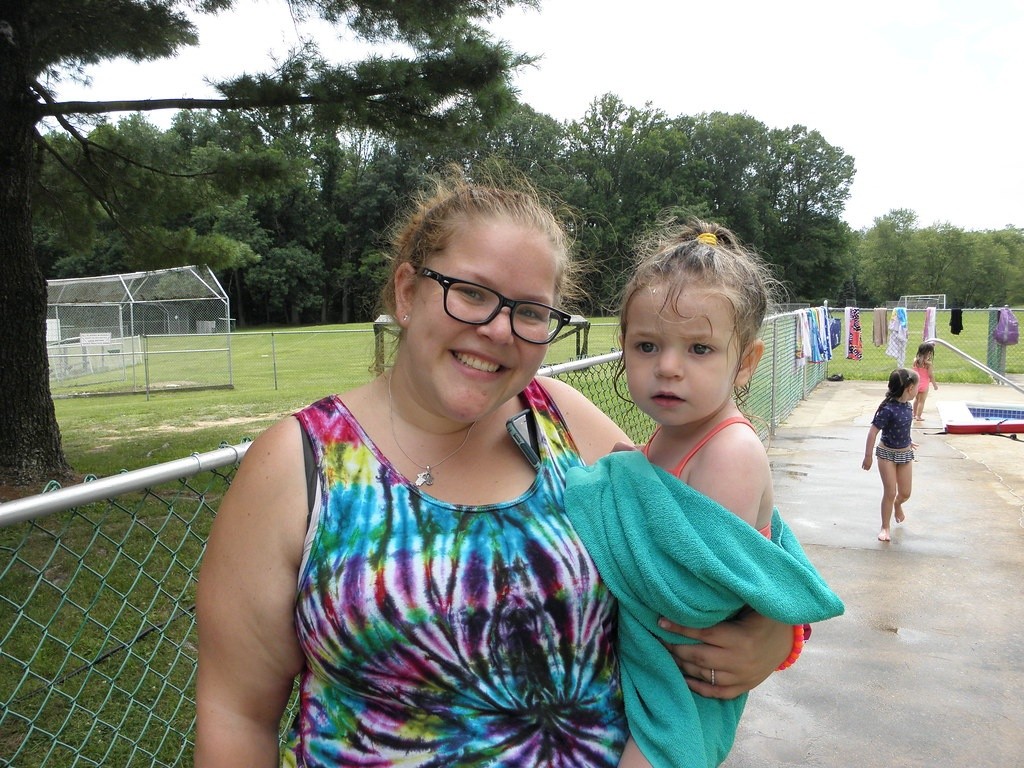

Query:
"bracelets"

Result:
[[773, 625, 806, 671]]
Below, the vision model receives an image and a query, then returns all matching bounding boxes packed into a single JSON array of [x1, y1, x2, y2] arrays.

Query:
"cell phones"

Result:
[[507, 409, 542, 473]]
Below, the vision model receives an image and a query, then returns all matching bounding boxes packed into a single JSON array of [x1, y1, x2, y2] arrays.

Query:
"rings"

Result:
[[711, 668, 715, 686]]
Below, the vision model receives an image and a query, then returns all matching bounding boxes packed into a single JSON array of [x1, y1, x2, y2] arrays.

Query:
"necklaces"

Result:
[[388, 369, 477, 486]]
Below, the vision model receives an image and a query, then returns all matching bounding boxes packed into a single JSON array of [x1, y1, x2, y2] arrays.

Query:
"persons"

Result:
[[610, 215, 773, 768], [862, 369, 920, 542], [911, 342, 937, 421], [193, 182, 811, 768]]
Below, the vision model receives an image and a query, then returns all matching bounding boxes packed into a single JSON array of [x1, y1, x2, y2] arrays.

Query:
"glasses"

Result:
[[413, 260, 574, 344]]
